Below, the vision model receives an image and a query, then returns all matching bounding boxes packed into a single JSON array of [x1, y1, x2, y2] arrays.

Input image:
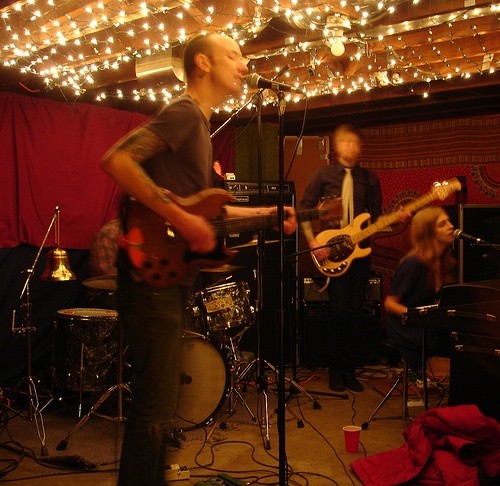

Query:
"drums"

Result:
[[117, 329, 231, 432], [52, 308, 121, 392], [186, 283, 255, 337]]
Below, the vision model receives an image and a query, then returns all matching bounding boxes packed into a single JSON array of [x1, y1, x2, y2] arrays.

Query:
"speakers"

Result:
[[302, 273, 384, 368]]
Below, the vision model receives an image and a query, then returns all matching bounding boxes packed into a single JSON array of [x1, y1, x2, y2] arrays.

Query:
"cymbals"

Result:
[[82, 274, 118, 291], [228, 238, 295, 249], [197, 264, 247, 272]]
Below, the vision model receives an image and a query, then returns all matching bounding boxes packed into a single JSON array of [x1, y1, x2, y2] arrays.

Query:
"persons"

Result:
[[86, 217, 122, 309], [384, 206, 456, 358], [98, 32, 297, 486], [299, 124, 411, 392]]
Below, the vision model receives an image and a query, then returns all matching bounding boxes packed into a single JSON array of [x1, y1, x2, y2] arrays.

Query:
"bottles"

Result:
[[213, 160, 221, 176]]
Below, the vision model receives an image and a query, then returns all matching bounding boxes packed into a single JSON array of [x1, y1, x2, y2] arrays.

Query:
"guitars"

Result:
[[123, 181, 344, 285], [310, 178, 462, 277]]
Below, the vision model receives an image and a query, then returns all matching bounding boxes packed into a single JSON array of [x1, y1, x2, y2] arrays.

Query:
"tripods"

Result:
[[0, 210, 58, 458], [57, 316, 181, 453], [206, 238, 351, 450]]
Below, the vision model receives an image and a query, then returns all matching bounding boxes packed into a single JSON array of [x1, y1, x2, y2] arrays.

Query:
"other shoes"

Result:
[[343, 374, 363, 392], [329, 375, 345, 391]]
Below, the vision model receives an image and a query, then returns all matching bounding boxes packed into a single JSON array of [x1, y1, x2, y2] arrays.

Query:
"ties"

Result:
[[340, 168, 354, 228]]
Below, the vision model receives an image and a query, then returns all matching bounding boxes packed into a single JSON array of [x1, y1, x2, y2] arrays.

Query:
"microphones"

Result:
[[452, 229, 487, 244], [246, 73, 303, 94]]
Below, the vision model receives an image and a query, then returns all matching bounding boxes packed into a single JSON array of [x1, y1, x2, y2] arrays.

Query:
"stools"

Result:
[[384, 339, 425, 424]]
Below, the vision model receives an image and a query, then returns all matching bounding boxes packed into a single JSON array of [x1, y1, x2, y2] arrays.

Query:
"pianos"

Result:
[[401, 278, 500, 486]]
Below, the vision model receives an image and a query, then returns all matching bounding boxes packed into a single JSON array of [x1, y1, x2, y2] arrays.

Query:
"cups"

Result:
[[343, 425, 361, 453]]
